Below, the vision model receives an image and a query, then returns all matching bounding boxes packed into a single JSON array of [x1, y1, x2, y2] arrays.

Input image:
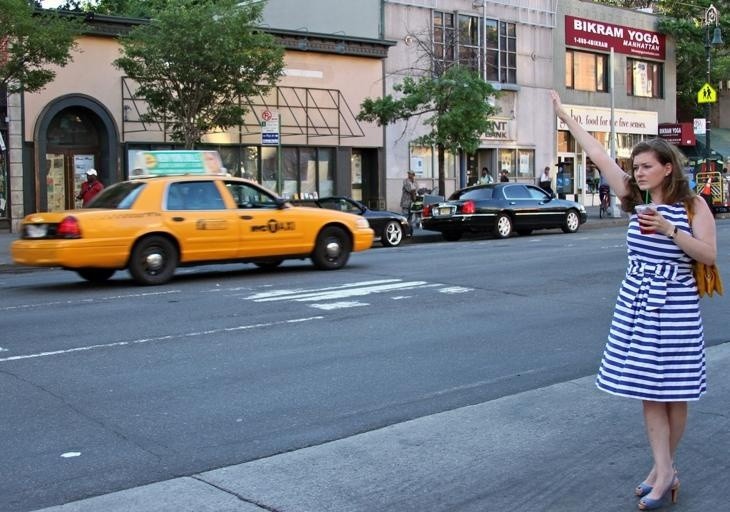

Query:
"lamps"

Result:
[[331, 31, 347, 54], [295, 27, 311, 50]]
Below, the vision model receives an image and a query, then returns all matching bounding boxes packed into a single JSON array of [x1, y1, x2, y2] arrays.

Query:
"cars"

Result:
[[11, 149, 383, 284], [420, 182, 589, 237], [295, 193, 415, 247]]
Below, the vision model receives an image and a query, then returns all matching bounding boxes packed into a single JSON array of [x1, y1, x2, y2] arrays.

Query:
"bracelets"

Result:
[[666, 224, 678, 239]]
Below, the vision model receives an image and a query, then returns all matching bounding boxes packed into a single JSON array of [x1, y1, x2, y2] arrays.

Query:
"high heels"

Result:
[[636, 464, 678, 494], [639, 471, 680, 510]]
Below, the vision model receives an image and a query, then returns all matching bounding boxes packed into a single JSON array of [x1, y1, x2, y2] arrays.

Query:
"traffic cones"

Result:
[[700, 176, 714, 195]]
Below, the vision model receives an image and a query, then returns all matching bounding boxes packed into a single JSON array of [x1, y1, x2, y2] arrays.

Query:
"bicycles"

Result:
[[595, 185, 611, 218]]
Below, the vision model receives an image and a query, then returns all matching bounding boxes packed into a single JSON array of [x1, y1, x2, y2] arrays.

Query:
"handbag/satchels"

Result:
[[693, 259, 725, 299]]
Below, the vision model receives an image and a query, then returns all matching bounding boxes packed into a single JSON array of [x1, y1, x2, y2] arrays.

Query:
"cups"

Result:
[[283, 192, 318, 200], [635, 204, 656, 237]]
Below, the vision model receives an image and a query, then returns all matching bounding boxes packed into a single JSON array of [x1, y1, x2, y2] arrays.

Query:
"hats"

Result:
[[85, 168, 97, 176]]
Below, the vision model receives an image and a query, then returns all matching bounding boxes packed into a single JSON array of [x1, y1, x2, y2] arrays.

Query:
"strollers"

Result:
[[407, 188, 435, 229]]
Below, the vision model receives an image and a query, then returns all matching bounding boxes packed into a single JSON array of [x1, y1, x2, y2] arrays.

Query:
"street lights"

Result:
[[703, 5, 723, 178]]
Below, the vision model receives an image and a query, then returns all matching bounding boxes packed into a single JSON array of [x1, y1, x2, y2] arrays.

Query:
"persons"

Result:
[[501, 170, 506, 176], [549, 89, 725, 511], [400, 170, 418, 216], [77, 168, 102, 208], [540, 165, 553, 183], [598, 172, 610, 207], [480, 165, 494, 183], [591, 164, 600, 193], [500, 169, 509, 182]]
